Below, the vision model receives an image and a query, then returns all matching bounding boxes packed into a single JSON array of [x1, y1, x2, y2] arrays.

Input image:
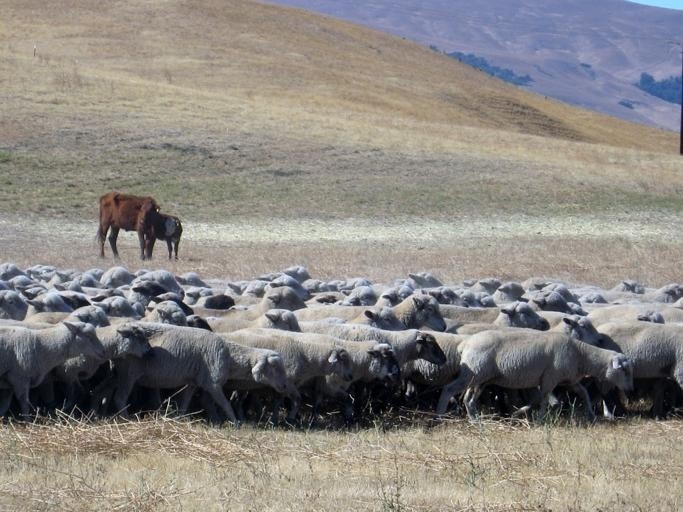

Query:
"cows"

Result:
[[157, 212, 184, 259], [96, 190, 161, 259]]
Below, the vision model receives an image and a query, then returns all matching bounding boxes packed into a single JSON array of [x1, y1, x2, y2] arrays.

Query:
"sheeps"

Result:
[[0, 263, 683, 427]]
